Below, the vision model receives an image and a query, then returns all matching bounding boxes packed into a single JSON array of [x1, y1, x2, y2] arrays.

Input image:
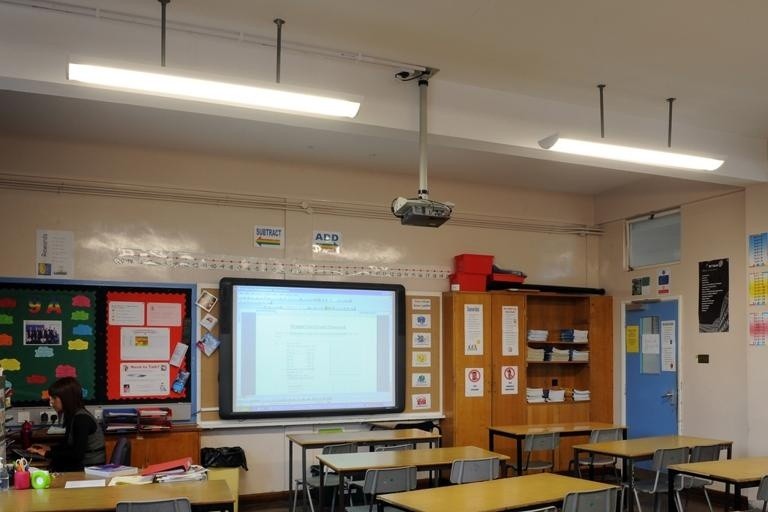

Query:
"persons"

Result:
[[26, 377, 106, 470]]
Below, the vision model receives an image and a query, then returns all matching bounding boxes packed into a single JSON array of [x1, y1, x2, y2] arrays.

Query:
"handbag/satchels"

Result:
[[201, 447, 248, 471]]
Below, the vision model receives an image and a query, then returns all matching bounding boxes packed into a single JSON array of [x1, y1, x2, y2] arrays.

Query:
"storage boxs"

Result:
[[453, 254, 494, 275], [449, 273, 487, 291]]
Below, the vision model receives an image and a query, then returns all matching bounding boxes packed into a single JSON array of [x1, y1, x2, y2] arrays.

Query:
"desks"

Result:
[[572, 435, 733, 512], [7, 444, 54, 474], [315, 446, 510, 511], [1, 479, 235, 512], [667, 457, 767, 512], [487, 422, 626, 475], [376, 473, 622, 512]]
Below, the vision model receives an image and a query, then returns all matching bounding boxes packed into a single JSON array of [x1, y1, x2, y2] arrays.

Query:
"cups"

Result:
[[14, 472, 30, 489]]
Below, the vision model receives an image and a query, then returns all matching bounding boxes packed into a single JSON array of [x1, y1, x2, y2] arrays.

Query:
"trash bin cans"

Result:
[[201, 445, 240, 512]]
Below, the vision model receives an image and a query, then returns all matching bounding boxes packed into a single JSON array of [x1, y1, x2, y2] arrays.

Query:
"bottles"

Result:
[[21, 428, 32, 450]]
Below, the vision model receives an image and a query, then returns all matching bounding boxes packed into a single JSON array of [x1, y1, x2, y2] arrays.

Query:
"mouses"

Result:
[[31, 445, 42, 450]]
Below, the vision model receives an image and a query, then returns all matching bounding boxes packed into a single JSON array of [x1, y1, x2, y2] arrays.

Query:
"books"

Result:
[[156, 464, 206, 483], [526, 346, 591, 361], [559, 329, 589, 343], [528, 330, 548, 342], [524, 385, 591, 402], [84, 463, 138, 478]]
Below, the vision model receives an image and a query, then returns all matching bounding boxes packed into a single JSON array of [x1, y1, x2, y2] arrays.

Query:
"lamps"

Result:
[[67, 0, 360, 120], [538, 86, 726, 173]]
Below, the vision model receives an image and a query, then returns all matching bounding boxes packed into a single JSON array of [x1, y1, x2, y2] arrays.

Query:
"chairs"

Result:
[[345, 465, 417, 512], [674, 444, 721, 512], [568, 428, 623, 486], [109, 438, 131, 467], [293, 442, 358, 512], [115, 498, 190, 512], [286, 428, 441, 512], [620, 446, 690, 512], [757, 476, 768, 510], [506, 432, 560, 479], [562, 489, 617, 512], [344, 444, 413, 507], [450, 457, 500, 485]]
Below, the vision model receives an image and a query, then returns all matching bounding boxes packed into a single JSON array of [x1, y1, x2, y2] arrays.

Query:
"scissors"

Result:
[[15, 458, 29, 472]]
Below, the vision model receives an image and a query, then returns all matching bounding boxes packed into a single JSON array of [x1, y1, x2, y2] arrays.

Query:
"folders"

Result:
[[142, 456, 193, 478], [105, 423, 138, 431], [104, 408, 138, 418], [140, 424, 171, 430]]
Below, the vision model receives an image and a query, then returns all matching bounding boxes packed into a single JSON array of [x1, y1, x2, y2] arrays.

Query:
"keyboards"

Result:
[[12, 447, 46, 461]]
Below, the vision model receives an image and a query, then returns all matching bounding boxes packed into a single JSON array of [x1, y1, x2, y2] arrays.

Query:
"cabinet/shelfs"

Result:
[[441, 292, 491, 480], [491, 291, 613, 478], [9, 424, 201, 470]]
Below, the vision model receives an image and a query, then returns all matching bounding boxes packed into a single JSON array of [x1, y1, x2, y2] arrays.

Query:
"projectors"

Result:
[[390, 195, 454, 228]]
